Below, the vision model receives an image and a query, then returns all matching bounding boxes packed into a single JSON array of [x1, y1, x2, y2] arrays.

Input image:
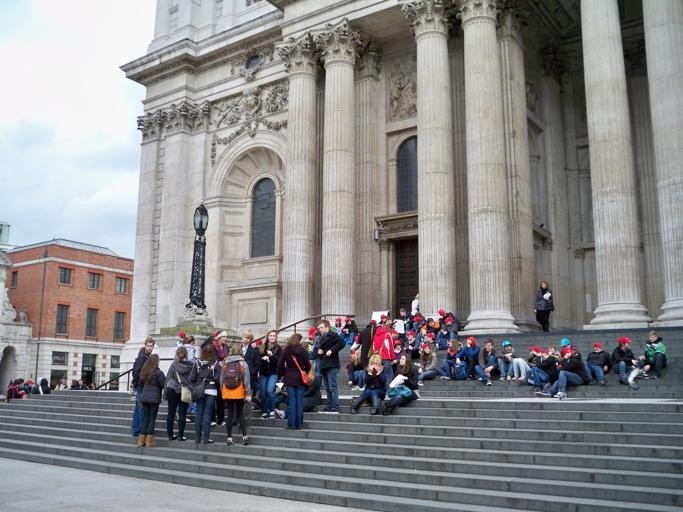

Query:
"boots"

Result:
[[146, 435, 158, 448], [371, 397, 381, 415], [350, 397, 365, 414], [136, 434, 146, 447]]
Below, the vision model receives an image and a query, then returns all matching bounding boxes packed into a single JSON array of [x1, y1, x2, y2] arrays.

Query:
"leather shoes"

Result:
[[169, 435, 176, 439], [203, 439, 214, 443], [178, 437, 187, 441]]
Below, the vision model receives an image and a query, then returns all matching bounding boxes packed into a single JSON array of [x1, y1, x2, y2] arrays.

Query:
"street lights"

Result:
[[176, 202, 214, 327]]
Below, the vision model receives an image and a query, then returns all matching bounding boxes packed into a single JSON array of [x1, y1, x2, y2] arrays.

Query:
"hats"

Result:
[[503, 341, 512, 347], [528, 336, 632, 356], [178, 332, 185, 337], [308, 327, 316, 333], [345, 317, 350, 322], [355, 310, 476, 348], [335, 317, 341, 321]]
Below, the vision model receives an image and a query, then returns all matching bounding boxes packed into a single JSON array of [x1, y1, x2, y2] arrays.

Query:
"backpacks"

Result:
[[223, 361, 243, 389]]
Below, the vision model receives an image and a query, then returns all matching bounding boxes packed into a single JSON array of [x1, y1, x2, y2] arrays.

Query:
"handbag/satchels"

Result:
[[545, 301, 554, 312], [192, 382, 204, 401], [180, 386, 193, 404], [297, 367, 316, 386]]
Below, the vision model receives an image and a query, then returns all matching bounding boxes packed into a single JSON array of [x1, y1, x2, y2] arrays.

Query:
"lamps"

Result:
[[373, 228, 380, 243]]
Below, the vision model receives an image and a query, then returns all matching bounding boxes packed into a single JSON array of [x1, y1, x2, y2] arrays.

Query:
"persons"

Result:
[[5, 377, 51, 402], [534, 282, 555, 332], [165, 319, 347, 446], [333, 308, 460, 416], [109, 378, 119, 390], [133, 337, 164, 447], [411, 292, 420, 317], [55, 375, 98, 390], [438, 330, 667, 398]]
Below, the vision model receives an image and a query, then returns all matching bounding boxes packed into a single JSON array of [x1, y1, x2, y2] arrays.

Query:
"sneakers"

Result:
[[478, 377, 568, 399], [242, 436, 249, 446], [440, 376, 450, 380], [318, 408, 328, 413], [324, 407, 340, 414], [260, 408, 286, 420], [417, 380, 425, 386], [226, 440, 232, 445], [169, 418, 237, 427]]
